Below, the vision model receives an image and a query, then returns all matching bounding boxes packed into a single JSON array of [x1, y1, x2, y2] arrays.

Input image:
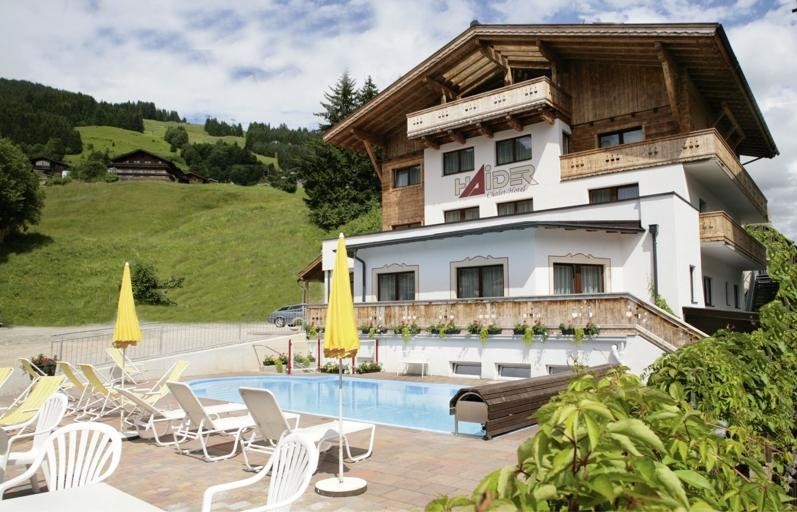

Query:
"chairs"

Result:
[[237, 386, 377, 472], [201, 433, 320, 512]]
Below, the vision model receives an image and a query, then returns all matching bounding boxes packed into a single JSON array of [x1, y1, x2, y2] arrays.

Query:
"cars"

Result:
[[268, 304, 306, 327]]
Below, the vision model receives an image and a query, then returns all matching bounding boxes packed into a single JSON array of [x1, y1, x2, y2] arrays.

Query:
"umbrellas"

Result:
[[110, 262, 140, 434], [321, 232, 360, 483]]
